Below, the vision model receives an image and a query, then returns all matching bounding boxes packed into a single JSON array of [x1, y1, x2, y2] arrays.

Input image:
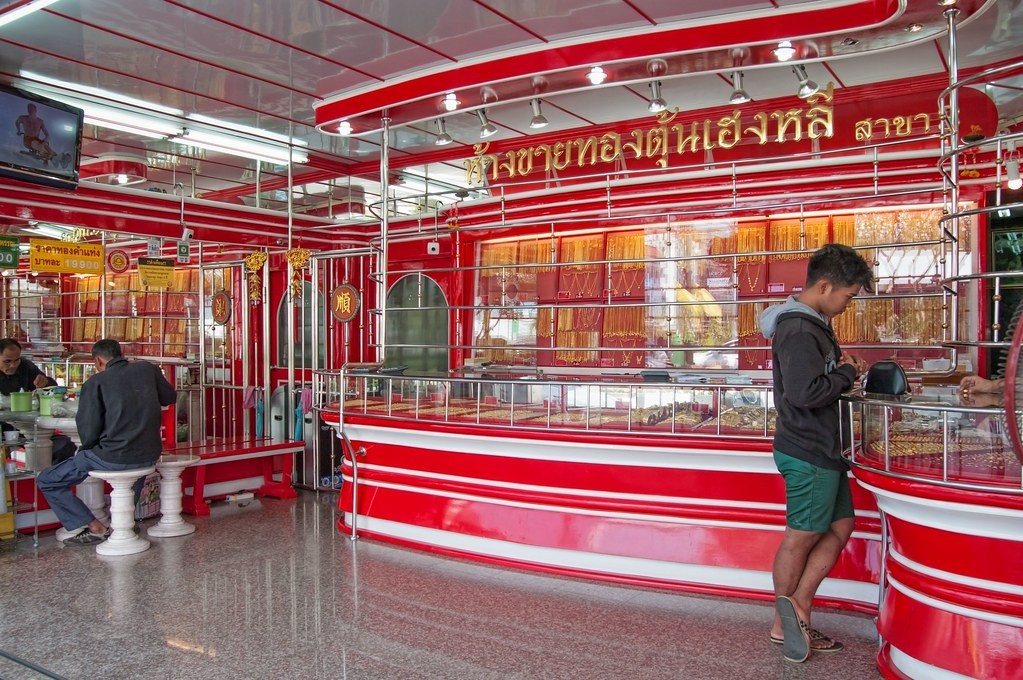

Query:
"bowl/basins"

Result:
[[4, 431, 20, 441]]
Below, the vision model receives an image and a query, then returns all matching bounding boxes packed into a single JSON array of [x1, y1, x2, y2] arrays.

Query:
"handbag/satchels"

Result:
[[861, 359, 911, 395]]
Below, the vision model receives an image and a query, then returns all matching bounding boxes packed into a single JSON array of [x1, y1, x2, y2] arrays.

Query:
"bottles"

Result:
[[5, 459, 15, 474], [64, 389, 80, 401], [19, 387, 39, 411]]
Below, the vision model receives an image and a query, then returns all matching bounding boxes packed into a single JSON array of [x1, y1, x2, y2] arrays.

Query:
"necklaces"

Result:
[[479, 209, 969, 367]]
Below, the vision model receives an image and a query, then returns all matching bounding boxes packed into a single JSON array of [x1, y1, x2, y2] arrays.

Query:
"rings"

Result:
[[870, 432, 1018, 471]]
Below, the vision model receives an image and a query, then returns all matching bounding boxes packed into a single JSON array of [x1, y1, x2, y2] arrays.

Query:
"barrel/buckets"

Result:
[[10, 393, 32, 411], [39, 395, 63, 416]]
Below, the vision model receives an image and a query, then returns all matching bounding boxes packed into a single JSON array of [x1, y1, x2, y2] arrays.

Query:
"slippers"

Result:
[[771, 628, 844, 652], [776, 596, 811, 662]]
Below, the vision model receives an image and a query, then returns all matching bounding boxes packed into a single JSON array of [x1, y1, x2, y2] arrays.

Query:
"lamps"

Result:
[[528, 98, 548, 128], [1004, 142, 1023, 189], [792, 64, 820, 98], [648, 81, 668, 112], [477, 108, 497, 138], [434, 116, 453, 145], [729, 70, 750, 104]]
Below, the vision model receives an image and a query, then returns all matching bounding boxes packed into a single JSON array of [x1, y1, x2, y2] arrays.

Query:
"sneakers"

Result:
[[63, 527, 110, 546], [134, 525, 141, 534]]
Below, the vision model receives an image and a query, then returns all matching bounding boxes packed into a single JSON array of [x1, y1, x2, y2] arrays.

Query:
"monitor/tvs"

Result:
[[0, 83, 84, 189]]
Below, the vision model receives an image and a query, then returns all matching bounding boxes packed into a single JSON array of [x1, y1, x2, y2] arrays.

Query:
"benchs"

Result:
[[160, 436, 306, 516]]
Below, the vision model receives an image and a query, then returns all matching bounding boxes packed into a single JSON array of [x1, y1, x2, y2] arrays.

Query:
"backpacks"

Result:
[[50, 435, 77, 466]]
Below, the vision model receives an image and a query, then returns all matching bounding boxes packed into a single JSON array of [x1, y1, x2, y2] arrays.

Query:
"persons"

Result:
[[17, 103, 72, 169], [959, 374, 1023, 396], [36, 339, 178, 546], [761, 244, 869, 663], [0, 338, 78, 466]]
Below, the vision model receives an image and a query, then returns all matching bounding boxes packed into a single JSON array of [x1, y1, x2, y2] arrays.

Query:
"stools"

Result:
[[86, 467, 150, 555], [148, 455, 201, 537]]
